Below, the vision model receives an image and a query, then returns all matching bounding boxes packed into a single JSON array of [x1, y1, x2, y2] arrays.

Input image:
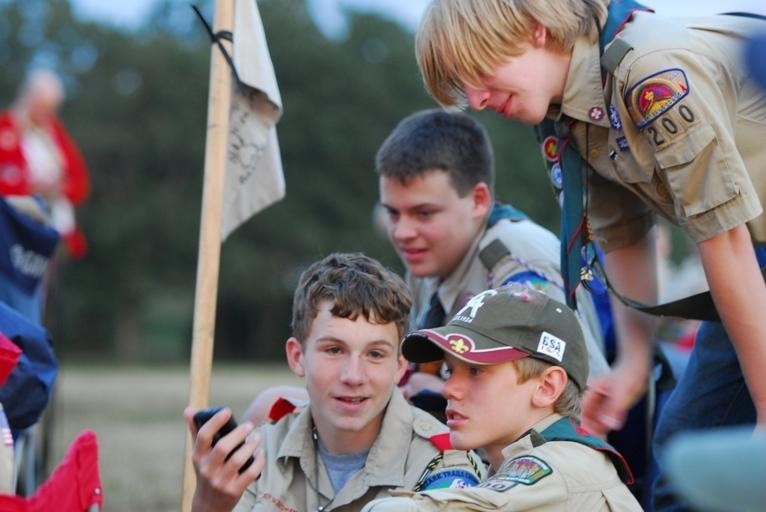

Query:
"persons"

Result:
[[184, 252, 486, 512]]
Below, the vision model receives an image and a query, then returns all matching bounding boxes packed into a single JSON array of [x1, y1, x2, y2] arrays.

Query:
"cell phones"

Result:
[[192, 406, 261, 484]]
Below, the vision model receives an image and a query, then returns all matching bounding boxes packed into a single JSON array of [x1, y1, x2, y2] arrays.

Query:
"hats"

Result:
[[401, 286, 589, 392]]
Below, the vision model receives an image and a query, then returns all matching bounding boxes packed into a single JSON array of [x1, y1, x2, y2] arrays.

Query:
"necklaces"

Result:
[[311, 428, 335, 511], [579, 123, 594, 280]]
